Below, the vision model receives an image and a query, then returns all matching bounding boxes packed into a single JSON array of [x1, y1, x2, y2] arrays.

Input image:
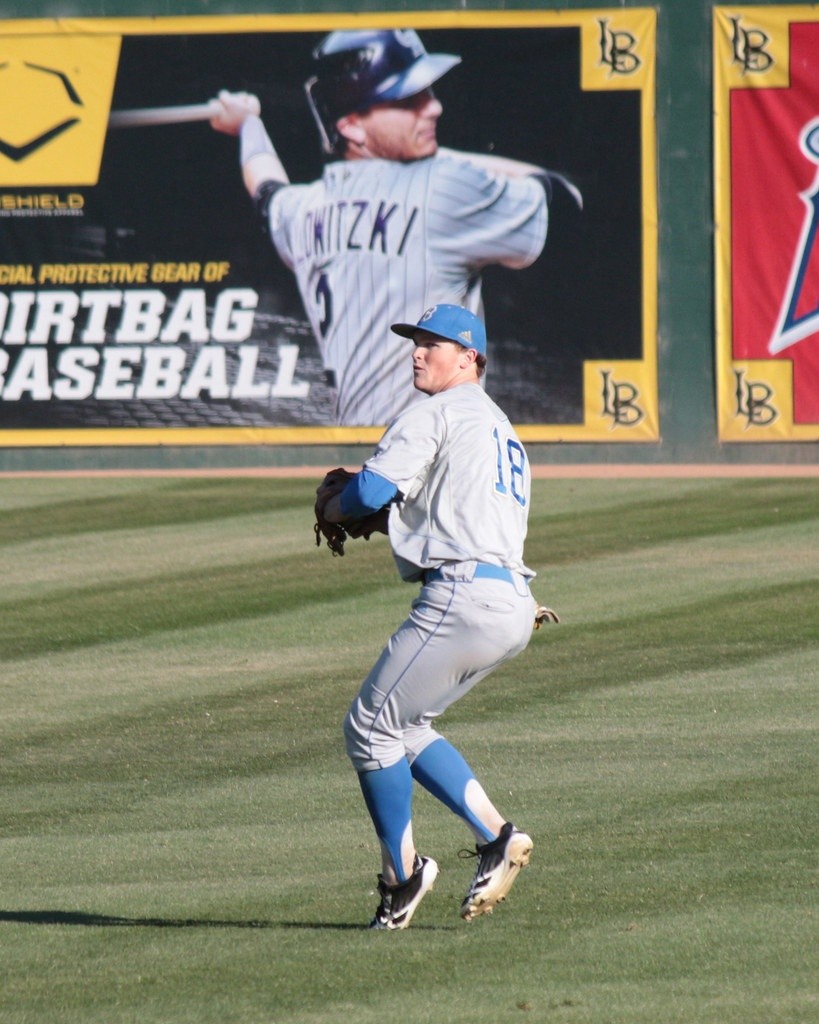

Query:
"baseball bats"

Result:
[[110, 94, 265, 127]]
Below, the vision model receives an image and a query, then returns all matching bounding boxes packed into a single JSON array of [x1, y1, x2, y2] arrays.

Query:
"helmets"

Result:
[[312, 26, 459, 122]]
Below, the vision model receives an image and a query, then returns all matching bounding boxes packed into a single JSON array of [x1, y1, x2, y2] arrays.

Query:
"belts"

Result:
[[421, 559, 528, 588]]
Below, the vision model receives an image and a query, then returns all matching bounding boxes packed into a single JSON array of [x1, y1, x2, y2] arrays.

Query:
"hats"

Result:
[[392, 304, 487, 359]]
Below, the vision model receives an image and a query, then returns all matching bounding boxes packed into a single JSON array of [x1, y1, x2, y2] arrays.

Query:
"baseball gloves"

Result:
[[317, 465, 384, 548]]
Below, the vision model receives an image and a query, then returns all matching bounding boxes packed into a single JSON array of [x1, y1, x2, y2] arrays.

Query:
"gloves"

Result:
[[535, 603, 561, 629]]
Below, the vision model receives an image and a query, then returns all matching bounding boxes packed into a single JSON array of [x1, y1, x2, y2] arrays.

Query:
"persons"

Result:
[[206, 25, 552, 428], [310, 303, 538, 931]]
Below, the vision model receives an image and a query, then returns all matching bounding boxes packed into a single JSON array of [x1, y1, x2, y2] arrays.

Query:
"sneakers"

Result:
[[456, 823, 533, 922], [371, 851, 441, 930]]
